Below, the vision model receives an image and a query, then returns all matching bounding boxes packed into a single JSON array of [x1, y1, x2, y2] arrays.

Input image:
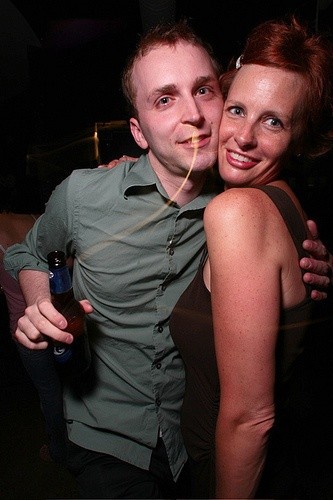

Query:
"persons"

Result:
[[3, 23, 333, 500], [93, 14, 332, 500]]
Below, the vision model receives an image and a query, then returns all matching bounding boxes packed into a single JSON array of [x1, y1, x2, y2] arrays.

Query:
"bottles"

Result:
[[47, 250, 92, 373]]
